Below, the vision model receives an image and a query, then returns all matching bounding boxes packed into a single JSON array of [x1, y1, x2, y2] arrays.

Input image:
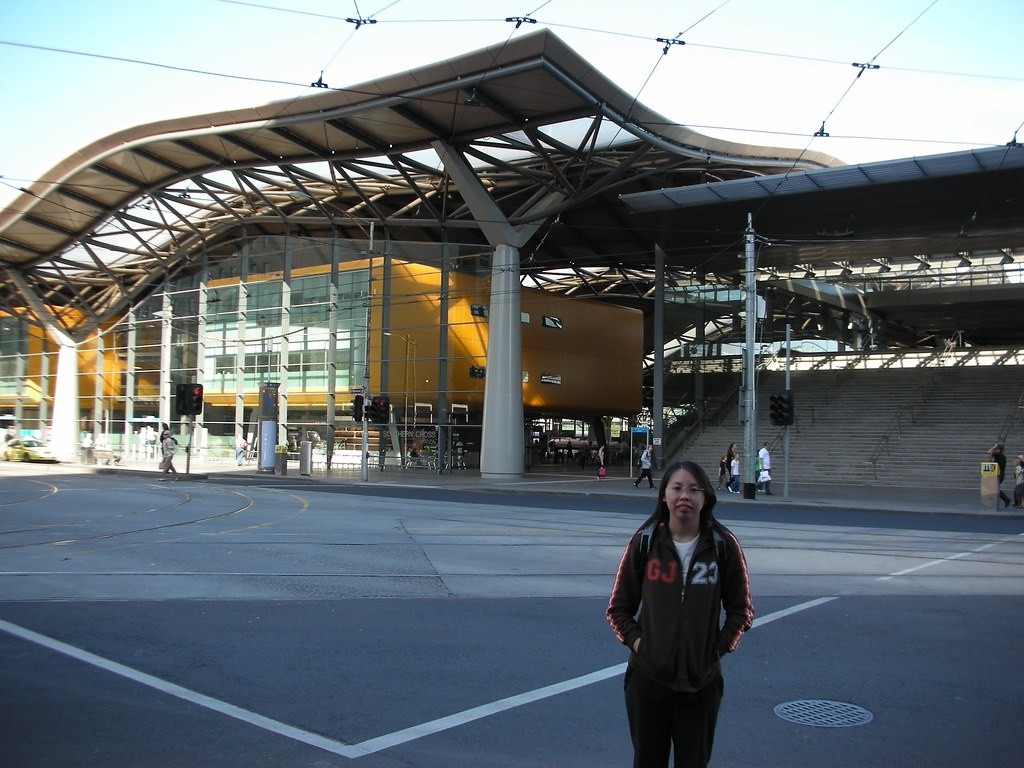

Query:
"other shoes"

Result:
[[716, 485, 740, 494], [649, 486, 656, 489], [1005, 499, 1011, 507], [175, 474, 181, 481], [631, 483, 638, 488], [765, 492, 772, 495], [158, 477, 165, 481], [758, 490, 764, 492]]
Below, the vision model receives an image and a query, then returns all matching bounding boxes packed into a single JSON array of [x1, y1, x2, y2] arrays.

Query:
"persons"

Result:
[[756, 442, 773, 495], [235, 435, 246, 466], [606, 461, 755, 768], [580, 441, 592, 471], [616, 441, 624, 465], [632, 444, 656, 488], [159, 423, 180, 481], [596, 444, 605, 480], [715, 442, 740, 493], [82, 433, 93, 446], [986, 444, 1012, 508], [1013, 454, 1024, 507], [407, 447, 418, 457]]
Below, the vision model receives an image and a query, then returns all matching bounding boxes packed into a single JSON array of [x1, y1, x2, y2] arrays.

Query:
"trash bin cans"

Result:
[[299, 440, 313, 475]]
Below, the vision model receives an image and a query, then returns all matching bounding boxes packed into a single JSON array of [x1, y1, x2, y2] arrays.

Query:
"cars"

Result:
[[0, 435, 57, 464]]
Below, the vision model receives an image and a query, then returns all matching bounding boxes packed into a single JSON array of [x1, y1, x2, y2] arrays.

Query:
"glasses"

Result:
[[667, 483, 704, 497]]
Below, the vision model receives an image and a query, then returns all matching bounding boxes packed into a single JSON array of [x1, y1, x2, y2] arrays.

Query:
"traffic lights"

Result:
[[187, 382, 204, 415], [349, 395, 363, 422], [369, 396, 389, 424], [768, 390, 794, 426]]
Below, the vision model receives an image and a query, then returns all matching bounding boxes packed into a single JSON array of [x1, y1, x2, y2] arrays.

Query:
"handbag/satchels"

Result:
[[598, 466, 607, 477], [758, 470, 771, 482], [159, 461, 165, 469], [166, 439, 178, 454]]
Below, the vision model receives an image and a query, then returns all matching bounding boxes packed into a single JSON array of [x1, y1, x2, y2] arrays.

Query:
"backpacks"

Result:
[[637, 451, 649, 467]]
